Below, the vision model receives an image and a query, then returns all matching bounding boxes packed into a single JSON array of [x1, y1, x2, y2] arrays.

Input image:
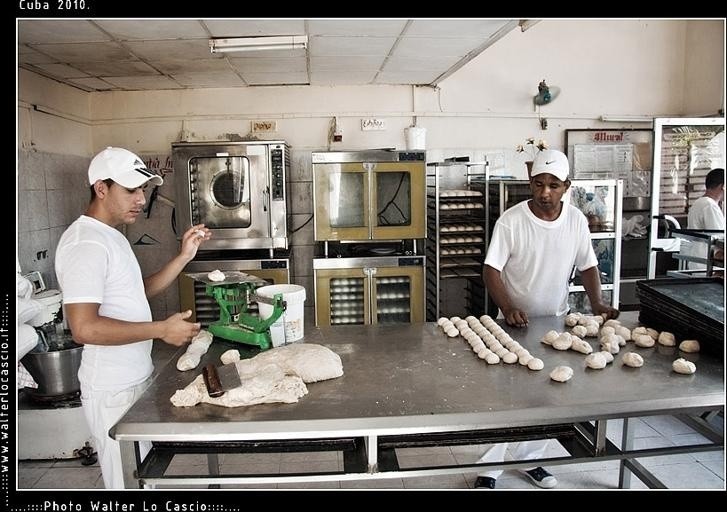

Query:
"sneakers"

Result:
[[518, 467, 558, 488], [474, 477, 496, 488]]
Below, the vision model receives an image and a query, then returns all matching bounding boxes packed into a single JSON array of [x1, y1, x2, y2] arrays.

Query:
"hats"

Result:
[[87, 146, 164, 190], [530, 150, 569, 182]]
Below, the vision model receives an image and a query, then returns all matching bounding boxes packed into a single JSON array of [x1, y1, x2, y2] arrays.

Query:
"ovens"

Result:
[[170, 142, 427, 323]]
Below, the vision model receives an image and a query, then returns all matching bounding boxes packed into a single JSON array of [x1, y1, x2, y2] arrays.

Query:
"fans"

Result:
[[534, 80, 559, 106]]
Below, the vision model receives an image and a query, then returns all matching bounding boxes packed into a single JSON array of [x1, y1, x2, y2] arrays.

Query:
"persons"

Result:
[[474, 150, 619, 488], [590, 186, 608, 222], [54, 146, 212, 490], [687, 169, 724, 277]]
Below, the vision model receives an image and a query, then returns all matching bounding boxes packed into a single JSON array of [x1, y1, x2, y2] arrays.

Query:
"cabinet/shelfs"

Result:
[[425, 159, 490, 323]]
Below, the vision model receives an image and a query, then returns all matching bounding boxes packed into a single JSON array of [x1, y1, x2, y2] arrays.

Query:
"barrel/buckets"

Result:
[[256, 283, 307, 349], [20, 319, 85, 396]]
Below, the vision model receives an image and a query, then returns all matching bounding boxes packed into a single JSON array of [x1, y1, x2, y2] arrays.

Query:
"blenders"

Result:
[[21, 272, 84, 403]]
